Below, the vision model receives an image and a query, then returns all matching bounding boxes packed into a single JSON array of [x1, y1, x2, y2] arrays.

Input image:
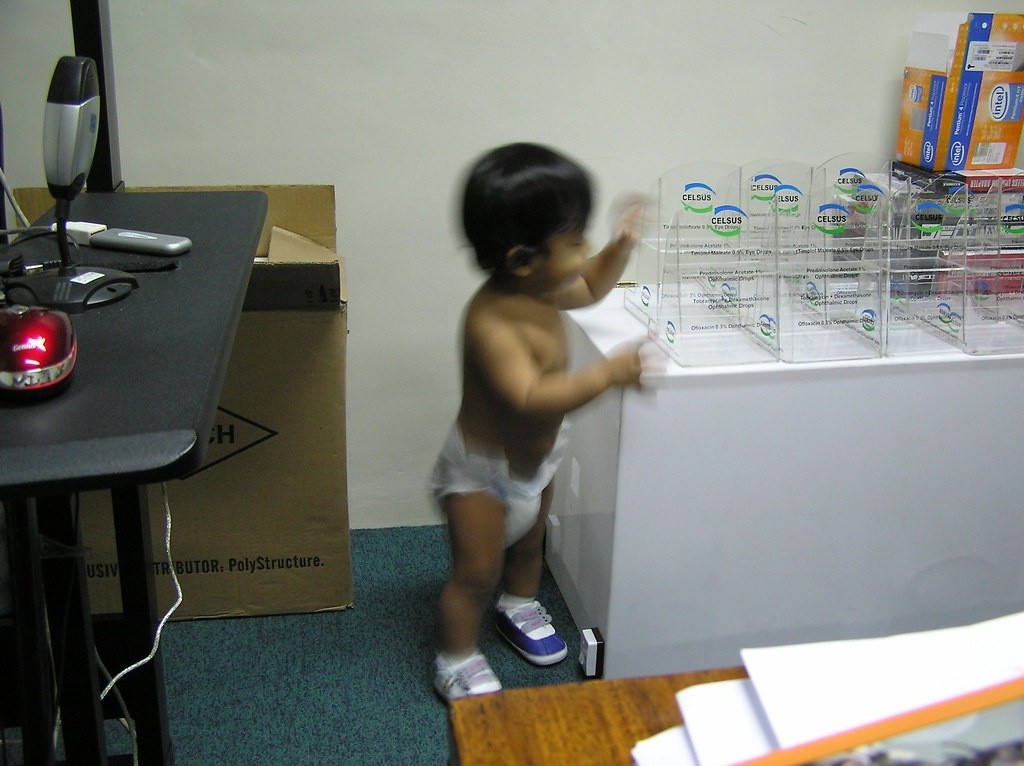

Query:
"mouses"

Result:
[[0, 305, 78, 389]]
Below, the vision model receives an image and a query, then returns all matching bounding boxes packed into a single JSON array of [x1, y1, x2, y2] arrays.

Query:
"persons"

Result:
[[434, 142, 670, 702]]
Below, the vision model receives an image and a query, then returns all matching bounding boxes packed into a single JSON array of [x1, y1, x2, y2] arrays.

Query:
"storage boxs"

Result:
[[831, 13, 1024, 294], [11, 184, 356, 623]]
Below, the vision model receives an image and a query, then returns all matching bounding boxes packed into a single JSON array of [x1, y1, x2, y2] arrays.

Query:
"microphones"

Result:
[[0, 56, 136, 313]]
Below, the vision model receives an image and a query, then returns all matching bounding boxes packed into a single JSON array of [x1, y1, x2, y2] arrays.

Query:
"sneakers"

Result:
[[492, 595, 568, 665], [433, 650, 502, 702]]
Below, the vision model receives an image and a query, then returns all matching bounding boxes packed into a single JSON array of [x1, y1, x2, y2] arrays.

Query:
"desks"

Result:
[[1, 190, 271, 766], [446, 667, 752, 765], [543, 285, 1023, 679]]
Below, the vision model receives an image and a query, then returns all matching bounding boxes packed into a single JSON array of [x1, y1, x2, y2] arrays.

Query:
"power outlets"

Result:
[[545, 514, 561, 552], [578, 628, 605, 677]]
